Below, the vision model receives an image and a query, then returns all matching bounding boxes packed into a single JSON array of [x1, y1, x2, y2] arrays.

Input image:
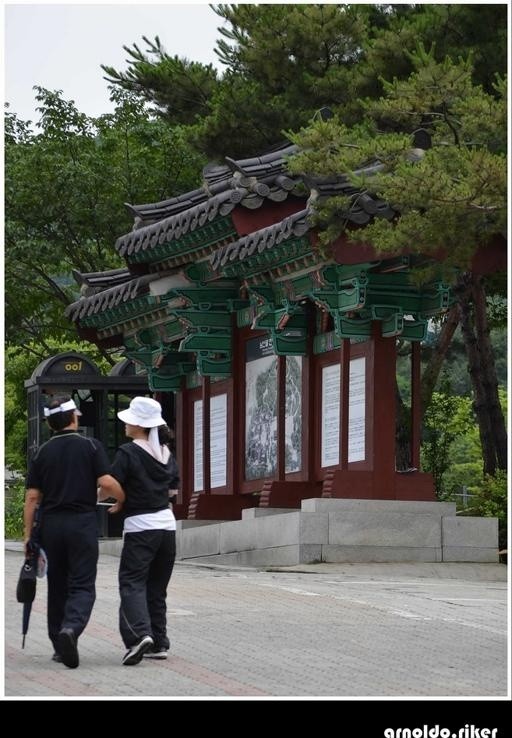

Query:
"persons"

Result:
[[98, 396, 181, 665], [22, 395, 127, 668]]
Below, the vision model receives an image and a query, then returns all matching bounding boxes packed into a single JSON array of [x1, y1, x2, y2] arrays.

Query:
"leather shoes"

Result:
[[52, 627, 80, 669]]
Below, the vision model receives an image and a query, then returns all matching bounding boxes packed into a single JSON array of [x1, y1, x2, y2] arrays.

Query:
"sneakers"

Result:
[[120, 634, 155, 667], [140, 645, 170, 660]]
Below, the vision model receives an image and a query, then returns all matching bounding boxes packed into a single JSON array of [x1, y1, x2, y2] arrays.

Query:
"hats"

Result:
[[115, 396, 167, 429], [43, 398, 83, 420]]
